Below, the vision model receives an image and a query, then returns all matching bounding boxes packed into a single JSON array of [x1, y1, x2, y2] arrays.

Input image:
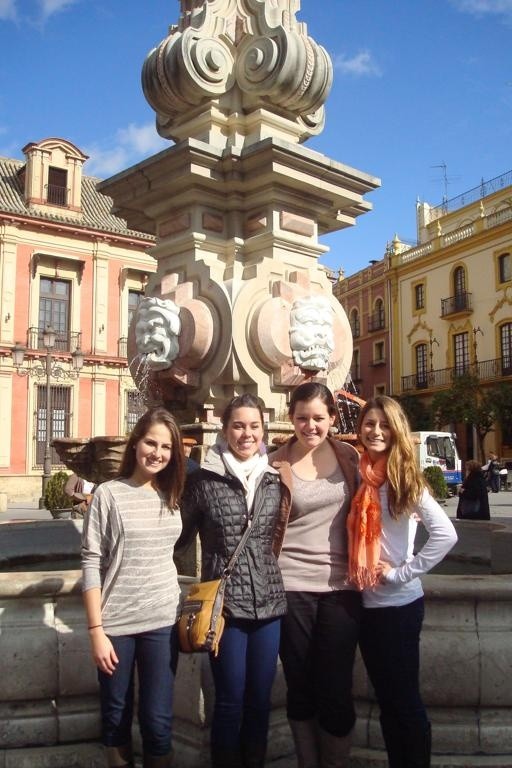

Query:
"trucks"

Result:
[[411, 429, 464, 492]]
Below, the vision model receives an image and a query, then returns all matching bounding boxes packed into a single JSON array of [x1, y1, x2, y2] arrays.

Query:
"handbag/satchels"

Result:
[[460, 498, 480, 516], [176, 578, 226, 658]]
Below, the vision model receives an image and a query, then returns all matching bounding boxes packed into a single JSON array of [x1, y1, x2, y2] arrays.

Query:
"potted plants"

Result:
[[423, 466, 448, 508], [44, 471, 73, 519]]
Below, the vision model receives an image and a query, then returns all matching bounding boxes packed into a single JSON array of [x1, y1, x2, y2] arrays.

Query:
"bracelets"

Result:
[[87, 624, 104, 629]]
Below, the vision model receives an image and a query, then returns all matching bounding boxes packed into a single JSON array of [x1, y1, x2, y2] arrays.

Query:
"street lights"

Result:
[[7, 321, 86, 511]]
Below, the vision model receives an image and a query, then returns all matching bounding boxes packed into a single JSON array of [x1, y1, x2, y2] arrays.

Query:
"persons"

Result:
[[81, 404, 186, 767], [84, 395, 291, 768], [457, 458, 491, 520], [64, 472, 97, 518], [268, 381, 363, 765], [487, 450, 501, 494], [347, 396, 461, 768]]
